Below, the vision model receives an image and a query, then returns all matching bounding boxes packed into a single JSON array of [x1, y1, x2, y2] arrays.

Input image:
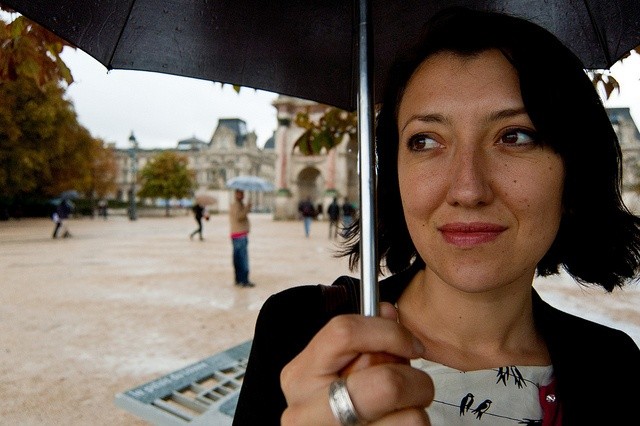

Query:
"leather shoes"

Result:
[[235, 280, 243, 286], [243, 282, 256, 287]]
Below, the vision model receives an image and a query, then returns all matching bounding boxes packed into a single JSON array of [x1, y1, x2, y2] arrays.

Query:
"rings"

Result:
[[328, 373, 360, 426]]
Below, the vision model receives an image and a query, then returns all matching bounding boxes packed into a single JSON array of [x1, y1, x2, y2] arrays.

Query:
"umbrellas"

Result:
[[224, 175, 276, 205], [47, 198, 63, 205], [55, 188, 81, 200], [0, 0, 640, 318]]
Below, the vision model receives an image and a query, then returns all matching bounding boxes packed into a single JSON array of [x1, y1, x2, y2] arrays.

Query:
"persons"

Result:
[[189, 197, 211, 241], [228, 187, 256, 289], [48, 195, 77, 239], [336, 195, 356, 236], [324, 194, 341, 239], [297, 192, 316, 241], [230, 1, 640, 426]]
